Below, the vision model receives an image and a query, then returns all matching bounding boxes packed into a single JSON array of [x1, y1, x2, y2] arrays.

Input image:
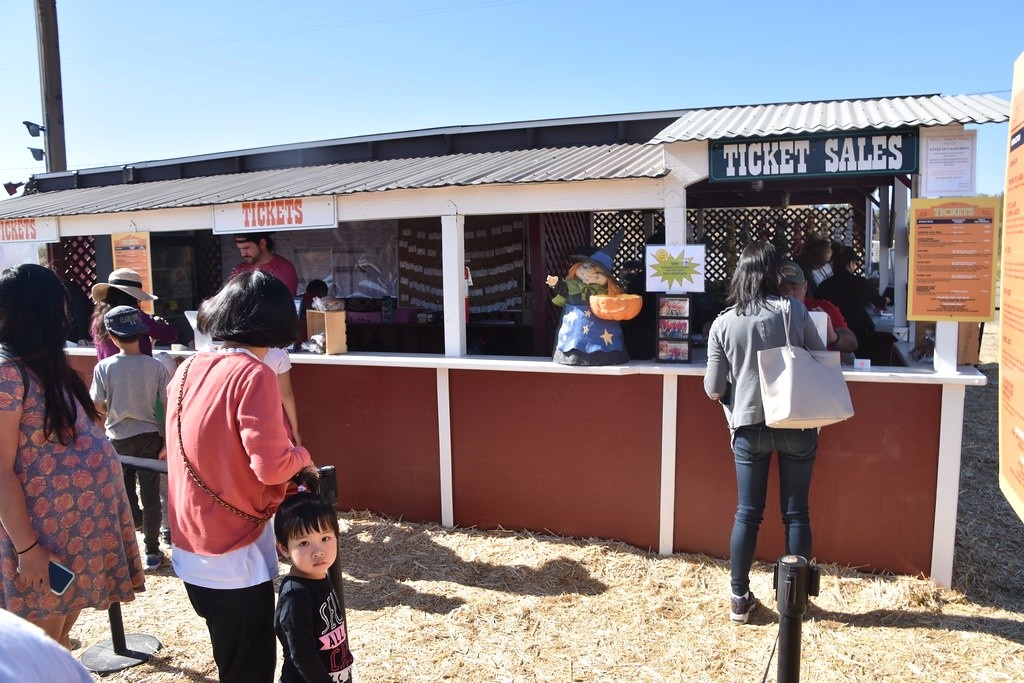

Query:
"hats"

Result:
[[234, 232, 275, 243], [91, 267, 158, 302], [844, 245, 862, 261], [773, 260, 805, 289], [103, 305, 150, 336]]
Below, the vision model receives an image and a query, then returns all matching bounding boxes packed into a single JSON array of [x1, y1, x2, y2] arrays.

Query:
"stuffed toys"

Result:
[[545, 229, 635, 365]]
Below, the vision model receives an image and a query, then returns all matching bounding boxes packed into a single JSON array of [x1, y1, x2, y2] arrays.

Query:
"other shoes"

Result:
[[163, 531, 171, 545], [147, 549, 165, 569], [134, 509, 144, 531]]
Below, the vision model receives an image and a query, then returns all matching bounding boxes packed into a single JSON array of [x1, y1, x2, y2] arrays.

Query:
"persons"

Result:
[[295, 278, 329, 347], [702, 243, 830, 625], [265, 347, 302, 445], [89, 305, 170, 569], [226, 233, 299, 301], [778, 238, 892, 365], [0, 263, 146, 650], [164, 268, 310, 683], [61, 279, 93, 347], [90, 269, 177, 361], [273, 493, 357, 683]]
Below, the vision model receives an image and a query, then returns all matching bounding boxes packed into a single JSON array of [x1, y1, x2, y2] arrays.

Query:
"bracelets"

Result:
[[16, 538, 39, 555], [829, 330, 840, 345]]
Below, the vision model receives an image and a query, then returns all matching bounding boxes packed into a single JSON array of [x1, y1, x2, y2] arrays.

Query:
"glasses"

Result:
[[818, 258, 834, 268]]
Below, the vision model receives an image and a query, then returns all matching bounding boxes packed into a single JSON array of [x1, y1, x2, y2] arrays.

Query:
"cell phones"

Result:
[[16, 560, 76, 596]]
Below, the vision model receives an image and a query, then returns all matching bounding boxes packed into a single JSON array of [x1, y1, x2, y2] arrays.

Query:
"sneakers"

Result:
[[732, 591, 756, 623]]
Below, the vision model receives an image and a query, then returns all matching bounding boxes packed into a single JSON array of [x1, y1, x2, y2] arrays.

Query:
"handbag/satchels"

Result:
[[756, 295, 855, 435]]
